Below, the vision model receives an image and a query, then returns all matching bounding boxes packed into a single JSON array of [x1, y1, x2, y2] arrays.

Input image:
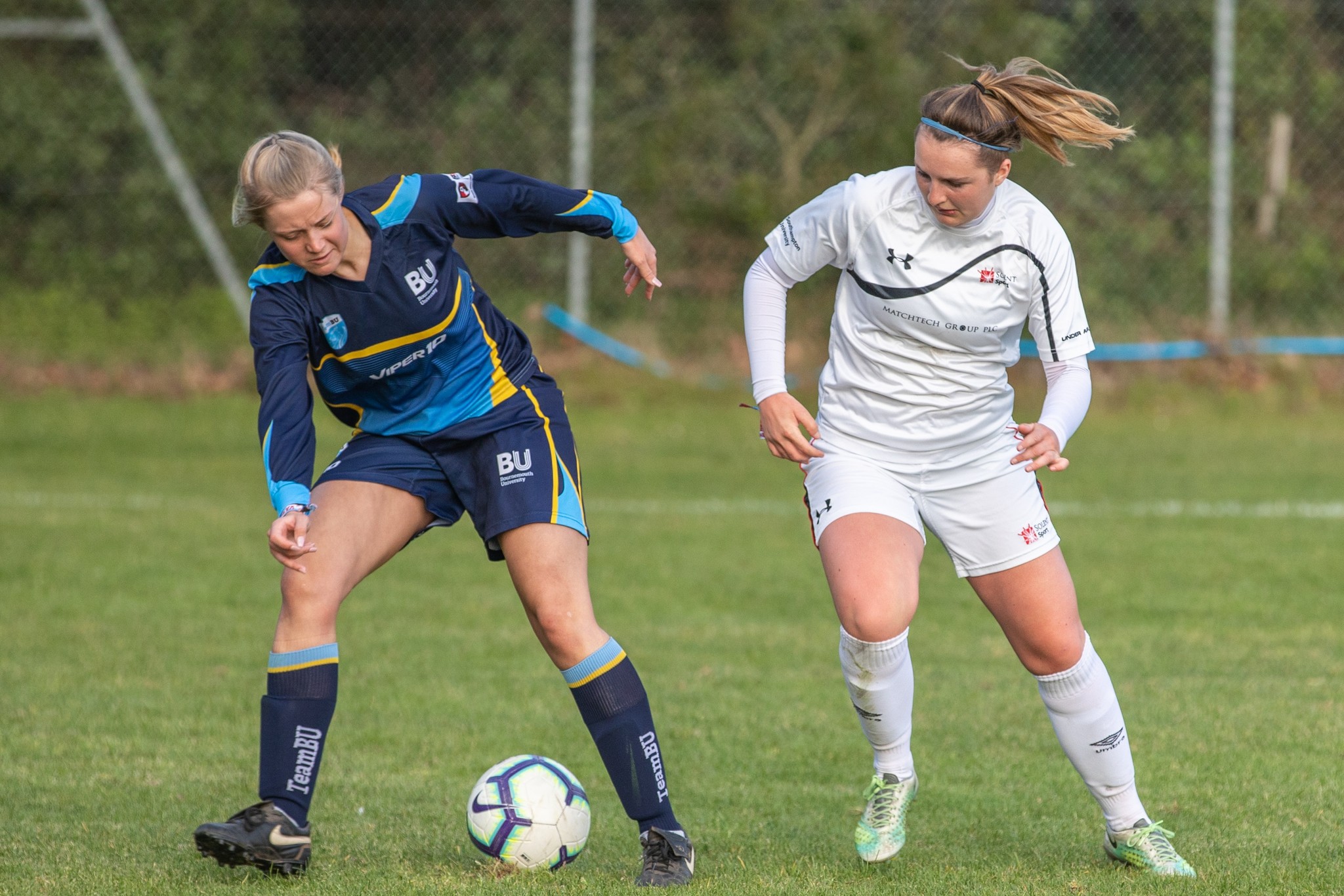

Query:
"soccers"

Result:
[[465, 754, 591, 872]]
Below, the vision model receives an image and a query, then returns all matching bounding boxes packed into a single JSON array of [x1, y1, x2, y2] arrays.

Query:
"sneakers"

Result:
[[1101, 819, 1194, 880], [855, 772, 919, 862], [636, 825, 695, 889], [193, 800, 312, 876]]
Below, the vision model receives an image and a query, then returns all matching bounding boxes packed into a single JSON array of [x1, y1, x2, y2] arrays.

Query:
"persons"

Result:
[[743, 52, 1196, 878], [195, 130, 696, 890]]
